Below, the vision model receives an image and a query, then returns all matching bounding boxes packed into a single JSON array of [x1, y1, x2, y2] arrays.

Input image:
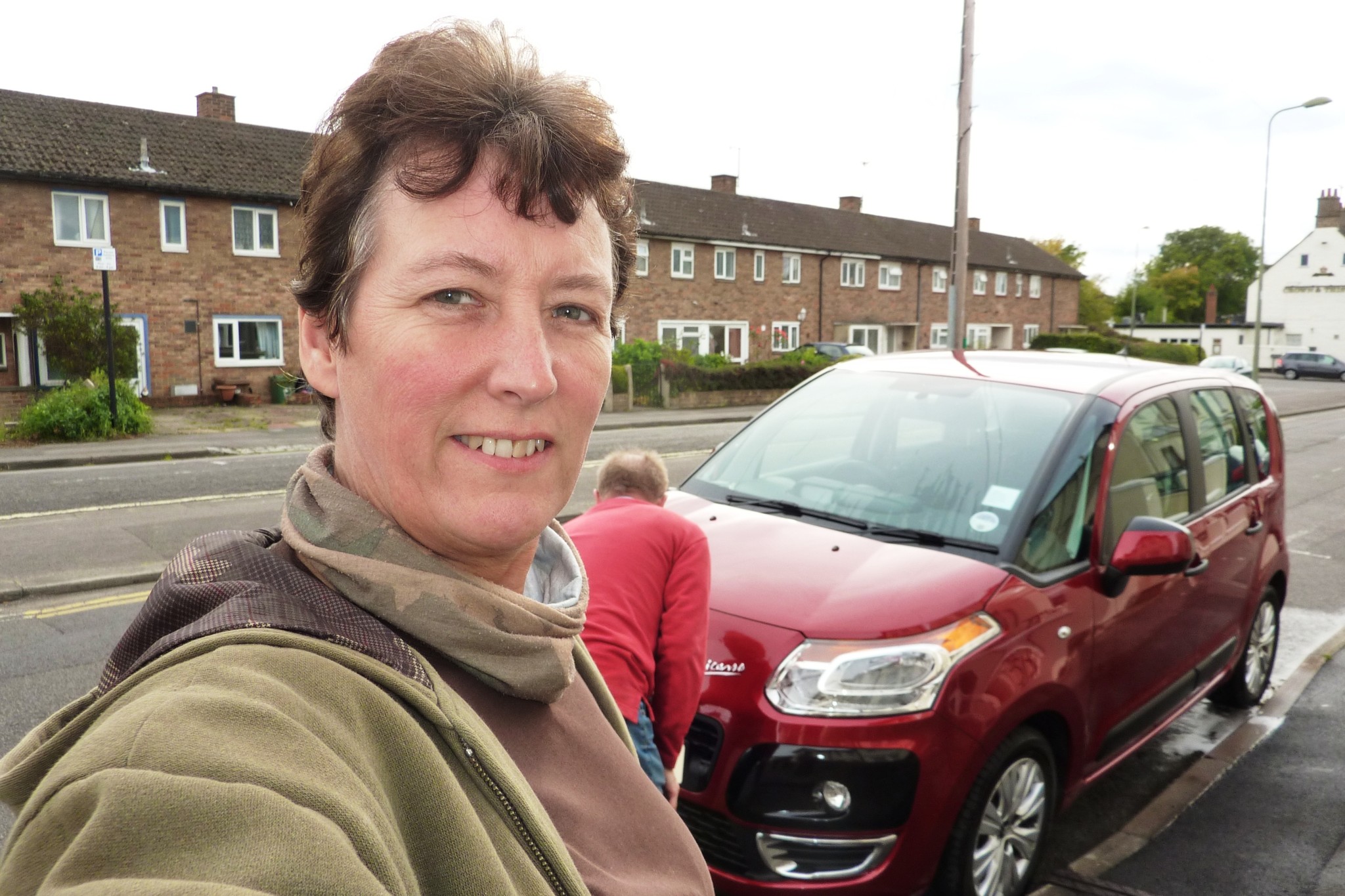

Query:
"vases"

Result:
[[216, 384, 237, 402]]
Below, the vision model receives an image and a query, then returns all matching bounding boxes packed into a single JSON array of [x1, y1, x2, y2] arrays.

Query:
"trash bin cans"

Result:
[[269, 375, 298, 403]]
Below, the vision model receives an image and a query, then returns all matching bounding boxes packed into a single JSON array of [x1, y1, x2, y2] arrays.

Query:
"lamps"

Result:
[[798, 306, 806, 323]]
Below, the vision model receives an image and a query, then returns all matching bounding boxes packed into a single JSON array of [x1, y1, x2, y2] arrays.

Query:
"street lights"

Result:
[[1249, 95, 1334, 384]]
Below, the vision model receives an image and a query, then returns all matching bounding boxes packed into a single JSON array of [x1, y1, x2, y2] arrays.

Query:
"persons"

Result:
[[0, 18, 716, 896], [561, 447, 712, 812]]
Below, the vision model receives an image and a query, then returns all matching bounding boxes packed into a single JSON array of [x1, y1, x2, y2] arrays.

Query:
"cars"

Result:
[[794, 342, 878, 362], [1199, 355, 1253, 380], [661, 347, 1291, 896], [1274, 352, 1345, 383]]
